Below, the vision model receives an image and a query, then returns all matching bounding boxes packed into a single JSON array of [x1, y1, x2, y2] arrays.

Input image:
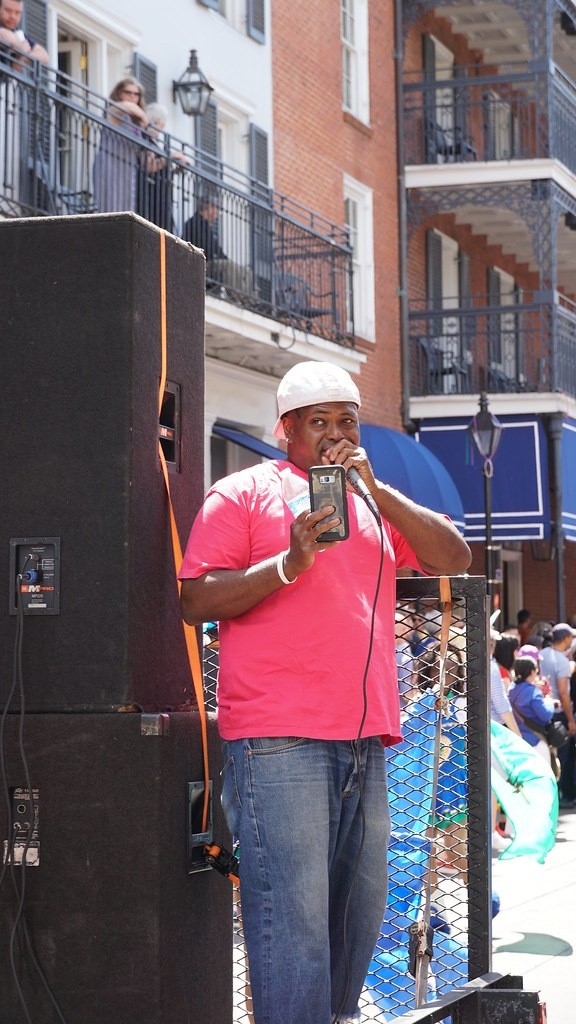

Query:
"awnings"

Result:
[[359, 422, 465, 537]]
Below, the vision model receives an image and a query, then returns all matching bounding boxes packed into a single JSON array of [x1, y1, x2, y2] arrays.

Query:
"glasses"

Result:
[[121, 90, 141, 97]]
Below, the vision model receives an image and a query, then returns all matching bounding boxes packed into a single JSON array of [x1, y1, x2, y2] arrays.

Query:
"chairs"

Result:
[[275, 271, 343, 344], [418, 336, 470, 395], [425, 118, 477, 163], [481, 366, 538, 393], [29, 158, 97, 214]]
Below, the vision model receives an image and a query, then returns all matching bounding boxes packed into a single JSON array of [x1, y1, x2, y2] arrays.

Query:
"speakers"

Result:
[[0, 211, 230, 1024]]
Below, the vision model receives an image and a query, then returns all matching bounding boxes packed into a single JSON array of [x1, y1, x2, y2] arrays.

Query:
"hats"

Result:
[[272, 360, 361, 439], [552, 623, 576, 639]]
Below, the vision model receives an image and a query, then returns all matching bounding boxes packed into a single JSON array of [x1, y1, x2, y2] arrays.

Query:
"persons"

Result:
[[137, 103, 192, 233], [0, 0, 49, 220], [92, 78, 149, 214], [177, 360, 472, 1024], [181, 195, 260, 312], [394, 599, 576, 929]]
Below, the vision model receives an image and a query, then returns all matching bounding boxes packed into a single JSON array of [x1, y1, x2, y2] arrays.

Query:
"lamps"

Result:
[[173, 49, 215, 116]]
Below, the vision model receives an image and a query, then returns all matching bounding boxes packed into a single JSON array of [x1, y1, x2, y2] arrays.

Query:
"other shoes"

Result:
[[491, 894, 500, 918], [496, 823, 511, 839], [428, 906, 450, 935]]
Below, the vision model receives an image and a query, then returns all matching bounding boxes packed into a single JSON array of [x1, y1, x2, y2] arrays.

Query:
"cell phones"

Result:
[[308, 464, 350, 542]]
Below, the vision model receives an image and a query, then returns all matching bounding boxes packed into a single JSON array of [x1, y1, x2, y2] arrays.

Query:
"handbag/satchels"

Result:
[[546, 722, 567, 746]]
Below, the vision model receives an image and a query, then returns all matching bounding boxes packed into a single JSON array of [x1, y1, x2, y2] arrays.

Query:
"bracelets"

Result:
[[277, 551, 297, 584]]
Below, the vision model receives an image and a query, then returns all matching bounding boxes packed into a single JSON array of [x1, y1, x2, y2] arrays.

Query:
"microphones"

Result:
[[330, 452, 382, 526]]
